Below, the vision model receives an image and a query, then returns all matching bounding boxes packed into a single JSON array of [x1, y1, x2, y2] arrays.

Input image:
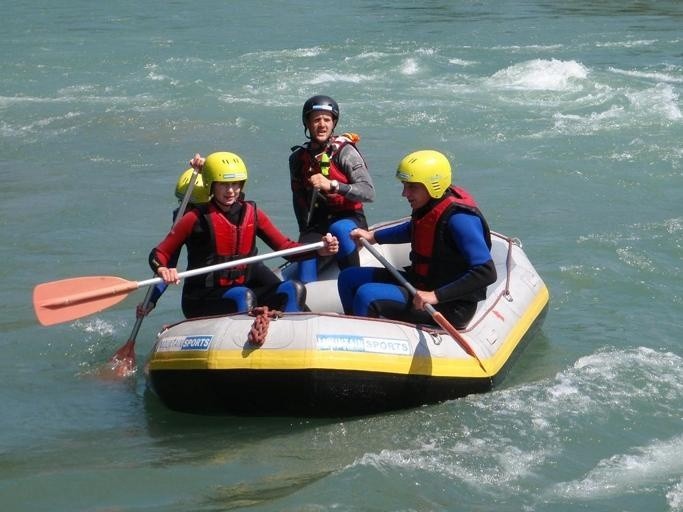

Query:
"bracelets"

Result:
[[327, 179, 338, 193]]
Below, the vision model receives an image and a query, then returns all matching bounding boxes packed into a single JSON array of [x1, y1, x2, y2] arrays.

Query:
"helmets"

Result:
[[175, 167, 214, 204], [302, 95, 338, 118], [201, 151, 247, 196], [395, 150, 451, 199]]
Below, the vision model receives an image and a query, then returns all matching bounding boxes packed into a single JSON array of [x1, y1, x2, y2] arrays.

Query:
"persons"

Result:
[[133, 153, 314, 317], [146, 151, 339, 317], [335, 148, 499, 330], [287, 95, 376, 284]]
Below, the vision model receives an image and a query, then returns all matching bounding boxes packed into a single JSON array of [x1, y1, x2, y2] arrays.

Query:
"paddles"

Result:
[[354, 235, 487, 372], [34, 241, 331, 325], [108, 164, 202, 375]]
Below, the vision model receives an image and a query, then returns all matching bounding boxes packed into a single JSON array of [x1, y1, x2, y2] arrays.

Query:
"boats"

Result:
[[145, 215, 550, 414]]
